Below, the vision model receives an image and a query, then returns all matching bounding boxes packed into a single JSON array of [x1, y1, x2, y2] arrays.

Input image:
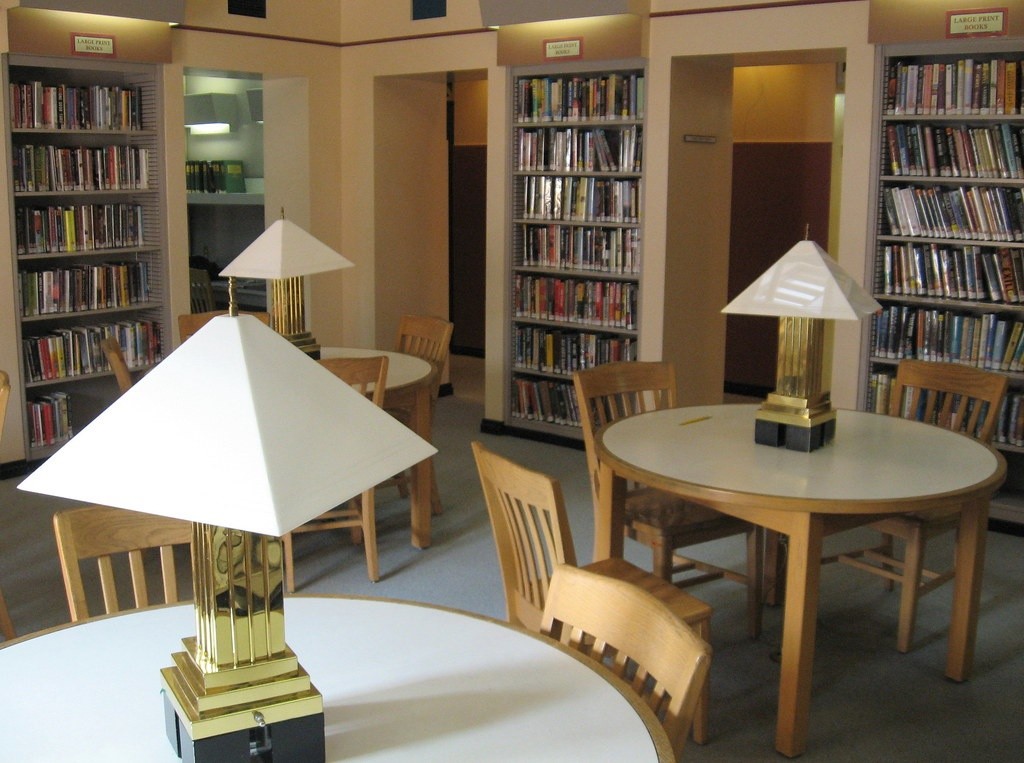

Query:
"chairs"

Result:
[[820, 357, 1009, 652], [571, 358, 763, 641], [373, 313, 454, 516], [470, 438, 714, 747], [101, 336, 132, 396], [277, 355, 380, 593], [539, 565, 711, 759], [52, 504, 195, 622], [178, 310, 270, 348], [188, 268, 216, 314]]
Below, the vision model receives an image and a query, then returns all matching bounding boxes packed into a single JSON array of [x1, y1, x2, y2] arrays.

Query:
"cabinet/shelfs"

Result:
[[0, 48, 173, 462], [855, 37, 1024, 538], [502, 57, 649, 440], [187, 191, 266, 309]]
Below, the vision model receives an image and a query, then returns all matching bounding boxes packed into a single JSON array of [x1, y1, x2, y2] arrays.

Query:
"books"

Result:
[[883, 186, 1024, 242], [514, 328, 637, 377], [886, 125, 1024, 179], [15, 204, 142, 254], [887, 61, 1024, 116], [26, 390, 74, 447], [16, 260, 149, 317], [870, 373, 1024, 446], [10, 142, 150, 191], [516, 274, 638, 330], [23, 320, 160, 382], [522, 224, 640, 277], [875, 306, 1024, 374], [511, 377, 603, 428], [184, 158, 248, 194], [520, 176, 644, 222], [8, 82, 144, 131], [517, 77, 646, 123], [517, 128, 644, 172], [882, 244, 1024, 307]]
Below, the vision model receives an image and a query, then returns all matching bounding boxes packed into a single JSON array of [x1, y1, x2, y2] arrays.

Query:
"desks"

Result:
[[318, 348, 436, 548], [0, 590, 677, 761], [593, 397, 1007, 758]]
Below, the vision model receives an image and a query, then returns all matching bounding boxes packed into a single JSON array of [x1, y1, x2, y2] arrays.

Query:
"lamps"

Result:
[[218, 205, 356, 361], [721, 222, 883, 453], [16, 276, 439, 763]]
